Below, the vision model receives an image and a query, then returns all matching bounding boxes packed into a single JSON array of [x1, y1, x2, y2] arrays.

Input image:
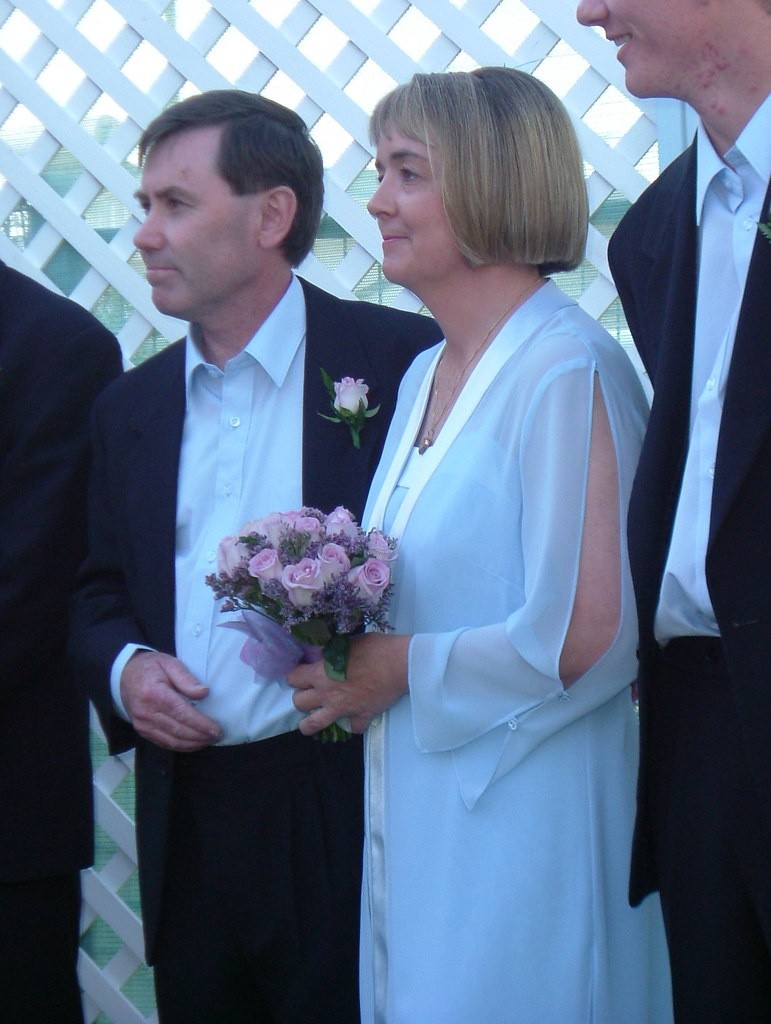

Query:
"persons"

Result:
[[573, 0, 771, 1023], [63, 91, 443, 1024], [287, 68, 665, 1023], [0, 263, 122, 1024]]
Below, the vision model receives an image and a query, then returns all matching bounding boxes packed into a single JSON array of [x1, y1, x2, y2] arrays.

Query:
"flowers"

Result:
[[316, 367, 381, 449], [205, 506, 398, 744]]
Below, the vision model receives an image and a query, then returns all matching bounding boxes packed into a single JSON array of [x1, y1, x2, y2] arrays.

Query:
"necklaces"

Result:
[[419, 277, 545, 455]]
